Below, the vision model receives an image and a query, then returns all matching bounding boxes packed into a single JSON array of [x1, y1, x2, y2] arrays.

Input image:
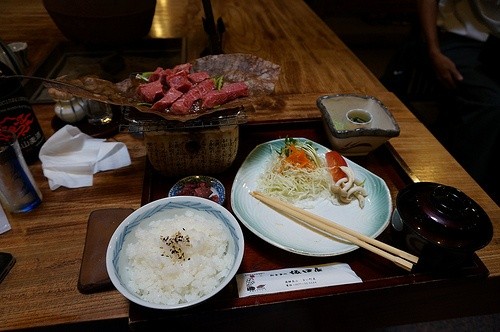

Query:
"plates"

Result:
[[51, 106, 122, 137], [169, 175, 225, 206], [231, 138, 393, 257], [106, 196, 244, 309]]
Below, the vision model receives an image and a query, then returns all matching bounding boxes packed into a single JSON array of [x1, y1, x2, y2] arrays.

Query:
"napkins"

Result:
[[38, 124, 132, 191]]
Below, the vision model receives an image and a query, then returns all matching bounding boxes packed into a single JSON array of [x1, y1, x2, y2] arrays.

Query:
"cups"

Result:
[[344, 109, 373, 130], [4, 42, 28, 74], [84, 99, 113, 124], [0, 130, 42, 214]]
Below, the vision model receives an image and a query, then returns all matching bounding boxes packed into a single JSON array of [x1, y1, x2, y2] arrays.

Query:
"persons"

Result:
[[419, 0, 500, 172]]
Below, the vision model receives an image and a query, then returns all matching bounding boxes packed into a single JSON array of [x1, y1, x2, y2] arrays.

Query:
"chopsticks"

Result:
[[249, 191, 419, 272]]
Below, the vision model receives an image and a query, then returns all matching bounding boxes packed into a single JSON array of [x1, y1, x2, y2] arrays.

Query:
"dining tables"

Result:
[[0, 0, 500, 332]]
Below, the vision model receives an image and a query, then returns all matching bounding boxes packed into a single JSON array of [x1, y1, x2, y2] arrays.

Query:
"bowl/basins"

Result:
[[317, 93, 401, 156], [391, 182, 494, 266], [141, 123, 239, 177]]
